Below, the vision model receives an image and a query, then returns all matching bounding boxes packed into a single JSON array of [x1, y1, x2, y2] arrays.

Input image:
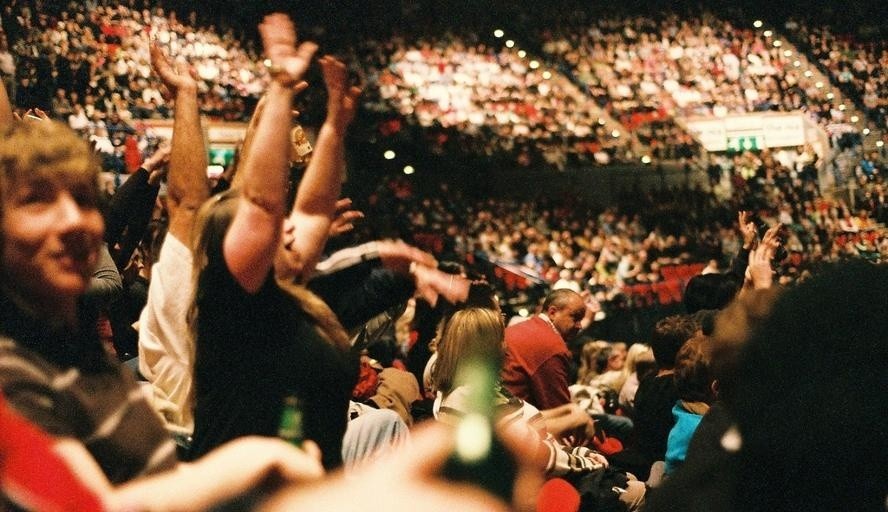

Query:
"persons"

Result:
[[1, 1, 888, 510]]
